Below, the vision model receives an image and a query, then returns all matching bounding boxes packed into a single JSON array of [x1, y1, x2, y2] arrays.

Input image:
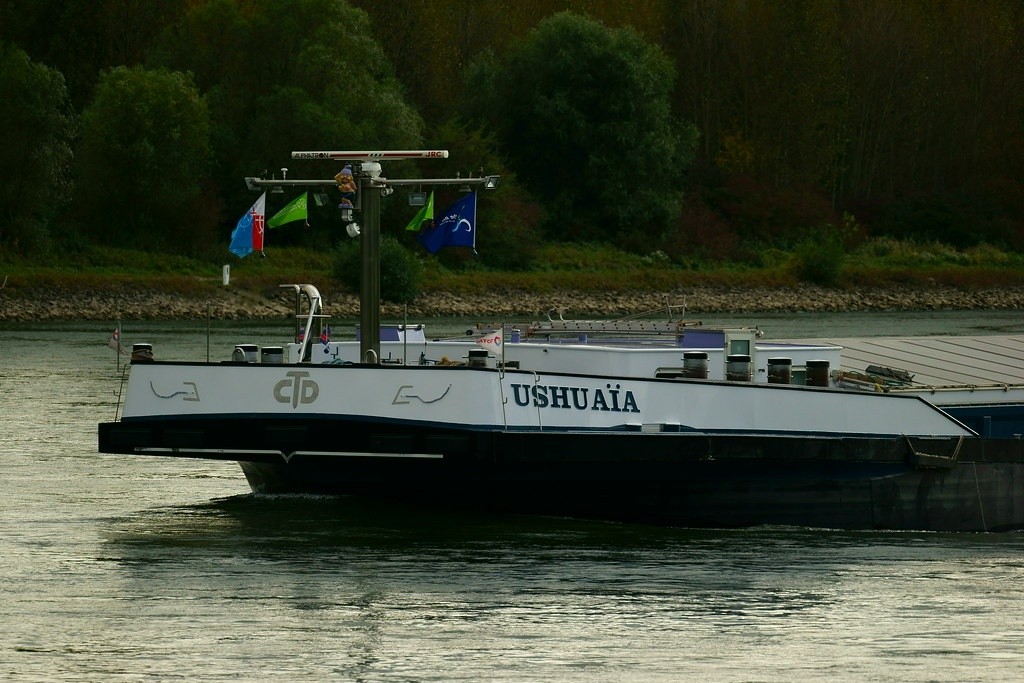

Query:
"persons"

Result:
[[334, 169, 357, 204]]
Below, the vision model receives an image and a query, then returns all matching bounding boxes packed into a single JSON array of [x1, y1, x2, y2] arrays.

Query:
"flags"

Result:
[[320, 326, 330, 344], [229, 192, 265, 258], [299, 328, 305, 341], [419, 193, 474, 252], [405, 191, 433, 231], [267, 192, 308, 229]]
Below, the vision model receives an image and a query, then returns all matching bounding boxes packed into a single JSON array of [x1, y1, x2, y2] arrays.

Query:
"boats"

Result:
[[98, 145, 1024, 531]]
